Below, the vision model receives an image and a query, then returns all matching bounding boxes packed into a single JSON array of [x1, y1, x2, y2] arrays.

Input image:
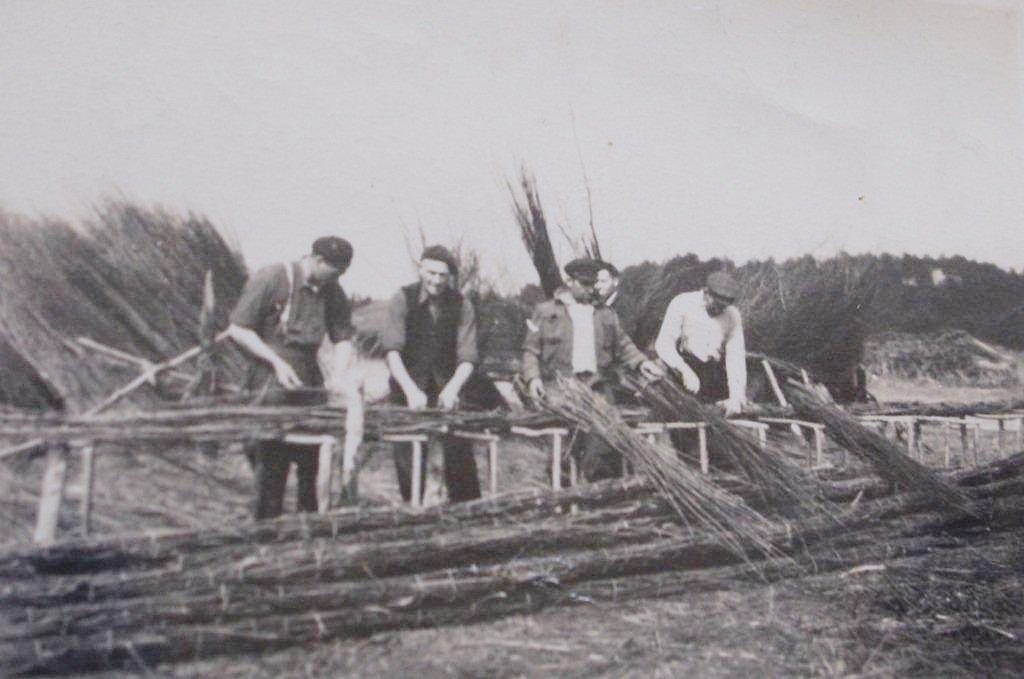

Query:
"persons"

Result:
[[383, 247, 481, 502], [656, 271, 748, 468], [228, 237, 354, 517], [522, 259, 661, 480]]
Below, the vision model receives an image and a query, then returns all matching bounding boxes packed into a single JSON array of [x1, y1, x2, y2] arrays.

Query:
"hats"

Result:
[[708, 272, 740, 299], [312, 236, 352, 262], [420, 245, 457, 273], [564, 259, 601, 277]]
